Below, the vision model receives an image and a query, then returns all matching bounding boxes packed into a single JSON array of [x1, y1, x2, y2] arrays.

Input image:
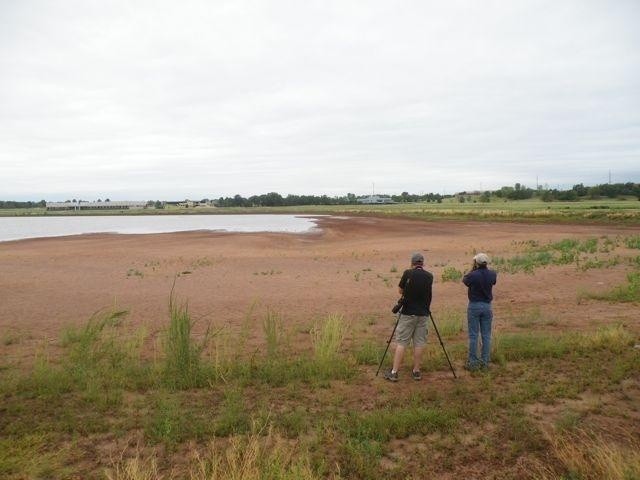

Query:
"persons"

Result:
[[383, 252, 433, 382], [462, 253, 497, 372]]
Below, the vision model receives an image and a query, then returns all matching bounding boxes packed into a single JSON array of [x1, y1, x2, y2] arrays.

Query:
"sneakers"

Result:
[[412, 369, 421, 380], [385, 370, 398, 381]]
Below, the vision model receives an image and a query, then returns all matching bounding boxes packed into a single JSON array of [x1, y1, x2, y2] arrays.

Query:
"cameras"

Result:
[[392, 299, 404, 313]]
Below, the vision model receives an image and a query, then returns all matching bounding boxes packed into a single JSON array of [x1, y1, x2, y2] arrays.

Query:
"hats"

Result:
[[474, 253, 489, 265], [413, 253, 424, 262]]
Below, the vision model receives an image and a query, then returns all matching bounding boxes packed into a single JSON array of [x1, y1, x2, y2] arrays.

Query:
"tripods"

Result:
[[375, 313, 457, 379]]
